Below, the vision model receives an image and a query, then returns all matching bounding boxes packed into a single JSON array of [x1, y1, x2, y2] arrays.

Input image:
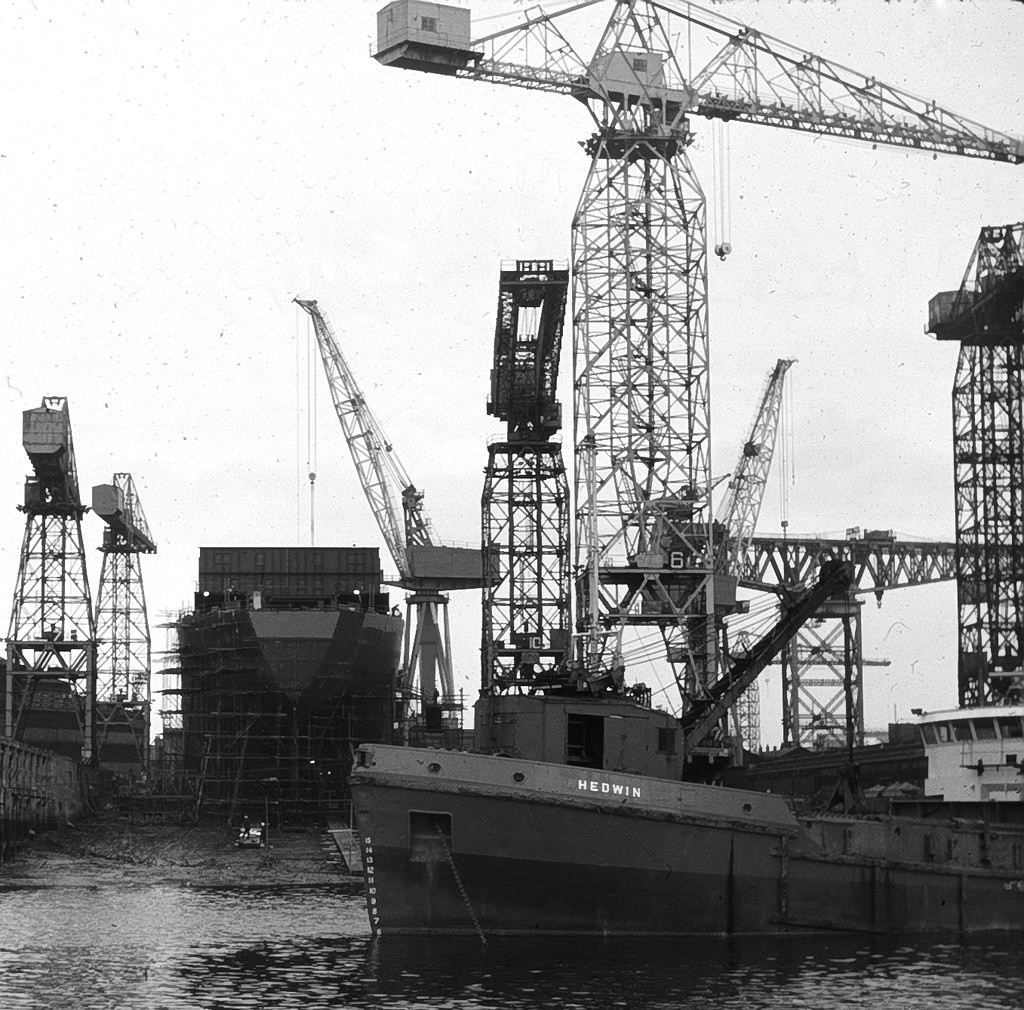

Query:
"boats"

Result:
[[340, 690, 1024, 939]]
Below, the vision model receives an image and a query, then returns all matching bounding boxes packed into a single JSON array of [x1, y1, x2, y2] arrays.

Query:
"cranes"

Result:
[[2, 0, 1024, 793]]
